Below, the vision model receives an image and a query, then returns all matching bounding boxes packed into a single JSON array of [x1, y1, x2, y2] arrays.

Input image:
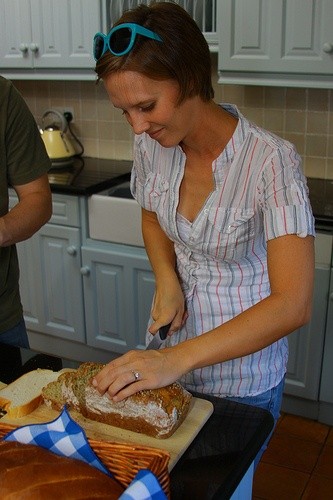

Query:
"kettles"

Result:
[[36, 110, 83, 166]]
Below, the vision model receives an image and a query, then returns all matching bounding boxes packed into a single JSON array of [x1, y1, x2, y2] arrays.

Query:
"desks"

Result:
[[0, 341, 277, 500]]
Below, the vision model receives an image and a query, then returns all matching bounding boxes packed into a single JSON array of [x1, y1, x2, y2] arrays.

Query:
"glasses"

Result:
[[92, 23, 162, 63]]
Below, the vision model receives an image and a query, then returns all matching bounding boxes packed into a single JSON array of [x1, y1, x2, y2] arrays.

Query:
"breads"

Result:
[[73, 363, 192, 439], [0, 441, 126, 500], [42, 360, 95, 411], [0, 369, 64, 417]]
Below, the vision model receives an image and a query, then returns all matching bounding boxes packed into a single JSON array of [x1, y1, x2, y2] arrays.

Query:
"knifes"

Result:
[[145, 321, 171, 351]]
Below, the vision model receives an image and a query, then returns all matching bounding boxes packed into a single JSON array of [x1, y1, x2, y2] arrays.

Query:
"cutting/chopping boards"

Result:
[[0, 367, 214, 474]]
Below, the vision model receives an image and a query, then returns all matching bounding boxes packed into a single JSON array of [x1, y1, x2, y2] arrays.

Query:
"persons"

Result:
[[92, 2, 315, 471], [0, 76, 53, 350]]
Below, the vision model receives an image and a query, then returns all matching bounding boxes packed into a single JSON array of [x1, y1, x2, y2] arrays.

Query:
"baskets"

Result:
[[0, 421, 172, 499]]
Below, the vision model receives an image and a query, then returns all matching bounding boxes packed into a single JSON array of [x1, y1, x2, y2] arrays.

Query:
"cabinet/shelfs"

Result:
[[216, 0, 333, 92], [7, 189, 86, 361], [80, 236, 158, 365], [281, 266, 333, 426], [0, 0, 108, 82]]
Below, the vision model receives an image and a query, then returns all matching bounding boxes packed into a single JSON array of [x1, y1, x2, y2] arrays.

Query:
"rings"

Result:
[[131, 370, 140, 381]]
